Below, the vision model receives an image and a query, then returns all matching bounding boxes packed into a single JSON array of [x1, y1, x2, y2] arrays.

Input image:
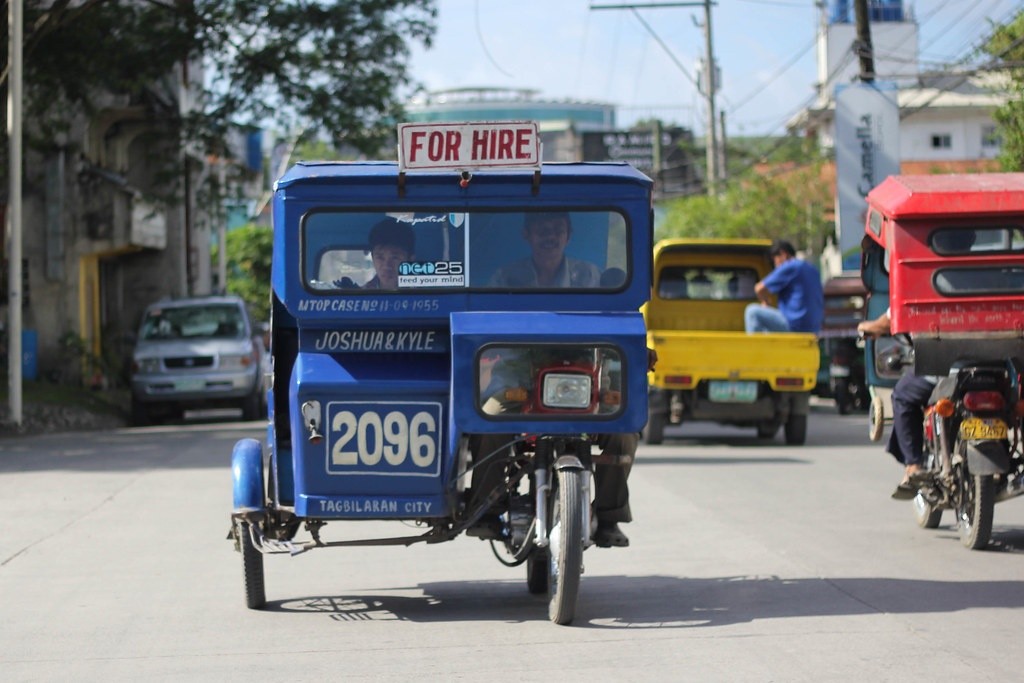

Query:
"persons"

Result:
[[857, 228, 1024, 500], [485, 212, 601, 291], [743, 241, 825, 333], [366, 219, 416, 290]]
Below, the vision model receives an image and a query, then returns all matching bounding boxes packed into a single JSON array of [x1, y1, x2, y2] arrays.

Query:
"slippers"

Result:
[[892, 470, 929, 501]]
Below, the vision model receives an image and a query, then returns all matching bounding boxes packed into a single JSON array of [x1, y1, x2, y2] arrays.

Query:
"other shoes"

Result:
[[596, 522, 630, 546], [466, 514, 503, 540]]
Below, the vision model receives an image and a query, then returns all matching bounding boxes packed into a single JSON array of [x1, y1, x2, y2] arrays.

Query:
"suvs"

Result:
[[132, 296, 271, 426]]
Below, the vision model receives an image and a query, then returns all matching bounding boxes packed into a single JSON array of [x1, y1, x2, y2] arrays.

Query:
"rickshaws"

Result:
[[815, 274, 872, 412], [228, 113, 657, 625], [859, 171, 1024, 551]]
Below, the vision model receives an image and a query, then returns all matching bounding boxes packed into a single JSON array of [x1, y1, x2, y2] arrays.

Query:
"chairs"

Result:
[[215, 321, 238, 337]]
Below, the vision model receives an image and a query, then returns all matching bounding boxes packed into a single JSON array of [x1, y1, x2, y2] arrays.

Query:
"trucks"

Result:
[[638, 236, 820, 446]]
